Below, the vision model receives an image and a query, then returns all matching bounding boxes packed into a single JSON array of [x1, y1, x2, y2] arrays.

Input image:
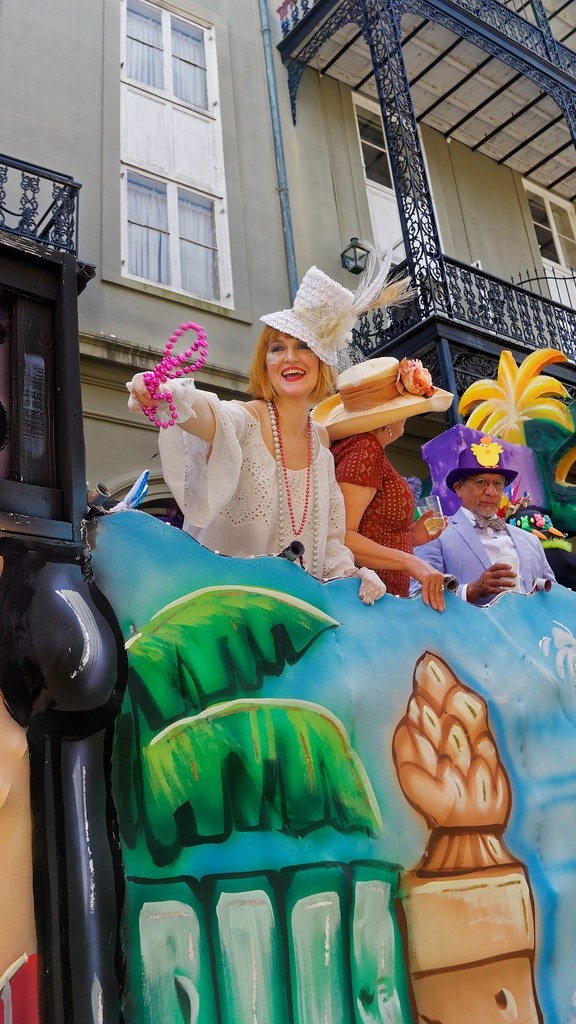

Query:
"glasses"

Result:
[[466, 478, 509, 490]]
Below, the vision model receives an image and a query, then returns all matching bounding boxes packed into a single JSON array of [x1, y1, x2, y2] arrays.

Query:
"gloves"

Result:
[[351, 567, 387, 606]]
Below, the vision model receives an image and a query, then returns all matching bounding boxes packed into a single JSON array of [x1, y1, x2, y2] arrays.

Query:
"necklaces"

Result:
[[265, 399, 318, 578], [141, 321, 208, 428]]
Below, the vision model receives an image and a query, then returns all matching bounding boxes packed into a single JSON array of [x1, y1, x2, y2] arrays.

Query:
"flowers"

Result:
[[396, 357, 435, 398]]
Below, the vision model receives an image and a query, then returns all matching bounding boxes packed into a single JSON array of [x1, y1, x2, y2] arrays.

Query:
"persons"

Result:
[[310, 355, 449, 611], [126, 269, 386, 606], [409, 437, 572, 607]]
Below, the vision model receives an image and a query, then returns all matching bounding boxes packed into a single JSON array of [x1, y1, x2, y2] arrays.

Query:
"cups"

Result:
[[416, 495, 445, 536]]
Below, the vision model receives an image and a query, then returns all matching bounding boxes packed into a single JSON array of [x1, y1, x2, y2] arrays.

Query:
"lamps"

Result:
[[340, 237, 370, 276]]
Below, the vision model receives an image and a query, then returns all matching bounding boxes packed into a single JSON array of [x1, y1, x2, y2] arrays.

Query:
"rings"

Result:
[[440, 585, 445, 592]]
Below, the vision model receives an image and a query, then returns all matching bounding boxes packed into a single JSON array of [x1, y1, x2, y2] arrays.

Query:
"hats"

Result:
[[259, 239, 417, 366], [445, 436, 519, 494], [309, 357, 454, 441]]
